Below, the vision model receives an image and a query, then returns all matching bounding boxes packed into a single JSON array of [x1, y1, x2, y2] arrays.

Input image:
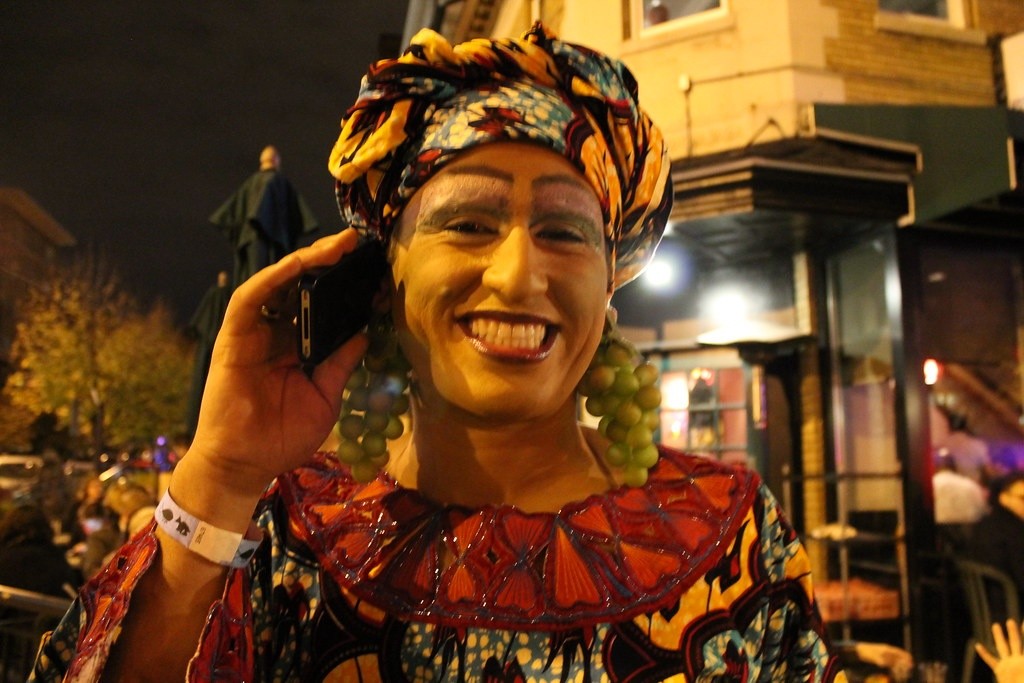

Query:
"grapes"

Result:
[[331, 321, 409, 482], [577, 313, 662, 487]]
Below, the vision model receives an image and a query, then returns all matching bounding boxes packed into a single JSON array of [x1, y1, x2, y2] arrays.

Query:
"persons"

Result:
[[935, 447, 988, 523], [968, 471, 1024, 626], [22, 20, 918, 683], [0, 454, 159, 600]]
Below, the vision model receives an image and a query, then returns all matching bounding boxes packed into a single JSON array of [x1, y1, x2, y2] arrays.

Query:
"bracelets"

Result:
[[154, 488, 265, 568]]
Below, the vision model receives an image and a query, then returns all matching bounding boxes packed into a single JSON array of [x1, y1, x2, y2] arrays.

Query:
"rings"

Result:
[[261, 304, 282, 319]]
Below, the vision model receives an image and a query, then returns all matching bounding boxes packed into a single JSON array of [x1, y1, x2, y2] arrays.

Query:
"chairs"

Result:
[[953, 560, 1020, 683]]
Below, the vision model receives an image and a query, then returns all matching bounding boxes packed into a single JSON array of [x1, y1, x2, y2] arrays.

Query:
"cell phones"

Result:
[[295, 234, 387, 367]]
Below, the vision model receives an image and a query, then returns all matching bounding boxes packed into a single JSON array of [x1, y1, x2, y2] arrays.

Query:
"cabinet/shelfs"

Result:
[[781, 471, 914, 657]]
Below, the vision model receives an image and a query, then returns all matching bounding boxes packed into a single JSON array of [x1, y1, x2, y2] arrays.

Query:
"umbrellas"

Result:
[[178, 147, 320, 450]]
